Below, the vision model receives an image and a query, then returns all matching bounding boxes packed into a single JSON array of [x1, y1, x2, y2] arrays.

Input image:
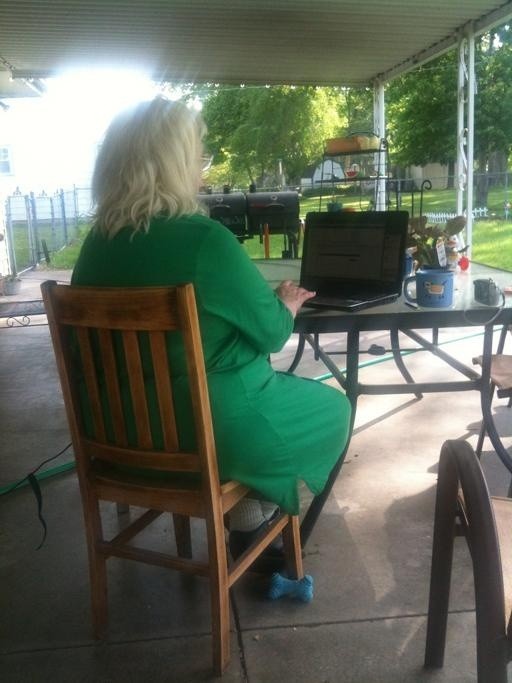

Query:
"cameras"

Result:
[[473, 279, 501, 306]]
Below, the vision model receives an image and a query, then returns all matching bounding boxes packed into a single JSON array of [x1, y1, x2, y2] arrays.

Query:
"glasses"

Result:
[[199, 154, 215, 172]]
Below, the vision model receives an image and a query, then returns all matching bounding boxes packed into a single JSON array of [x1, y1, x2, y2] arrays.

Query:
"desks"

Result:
[[253, 272, 512, 548]]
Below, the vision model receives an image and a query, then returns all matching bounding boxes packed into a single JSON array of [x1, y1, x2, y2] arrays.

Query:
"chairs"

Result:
[[41, 277, 306, 675]]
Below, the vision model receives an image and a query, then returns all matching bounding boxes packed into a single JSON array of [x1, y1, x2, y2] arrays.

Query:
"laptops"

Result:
[[293, 210, 409, 312]]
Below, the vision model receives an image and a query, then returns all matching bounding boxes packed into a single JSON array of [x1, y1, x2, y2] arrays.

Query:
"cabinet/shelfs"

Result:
[[312, 128, 391, 212]]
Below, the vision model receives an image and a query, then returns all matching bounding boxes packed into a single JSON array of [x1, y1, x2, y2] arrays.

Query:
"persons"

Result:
[[67, 99, 353, 575]]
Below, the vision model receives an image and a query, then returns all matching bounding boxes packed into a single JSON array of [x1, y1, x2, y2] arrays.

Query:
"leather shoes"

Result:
[[229, 521, 306, 566]]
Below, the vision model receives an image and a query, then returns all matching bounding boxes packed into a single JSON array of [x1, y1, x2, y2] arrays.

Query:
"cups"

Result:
[[403, 268, 454, 308]]
